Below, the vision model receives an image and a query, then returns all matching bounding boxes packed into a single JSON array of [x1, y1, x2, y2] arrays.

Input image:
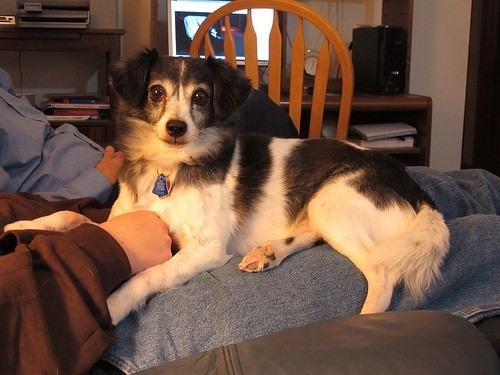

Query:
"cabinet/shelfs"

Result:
[[0, 26, 127, 144], [278, 93, 433, 168]]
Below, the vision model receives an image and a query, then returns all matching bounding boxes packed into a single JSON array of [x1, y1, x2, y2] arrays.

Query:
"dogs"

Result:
[[1, 45, 453, 328]]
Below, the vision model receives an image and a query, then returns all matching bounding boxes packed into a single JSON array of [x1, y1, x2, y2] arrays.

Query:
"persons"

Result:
[[1, 168, 500, 375], [0, 88, 126, 209]]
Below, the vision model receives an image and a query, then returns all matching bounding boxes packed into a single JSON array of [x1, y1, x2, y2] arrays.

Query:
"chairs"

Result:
[[188, 0, 354, 142], [126, 309, 500, 375]]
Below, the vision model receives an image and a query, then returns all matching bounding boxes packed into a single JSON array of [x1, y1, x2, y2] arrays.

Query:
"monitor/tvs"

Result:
[[172, 9, 248, 61]]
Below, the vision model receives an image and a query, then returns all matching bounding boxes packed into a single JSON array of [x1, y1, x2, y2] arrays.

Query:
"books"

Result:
[[41, 95, 113, 120]]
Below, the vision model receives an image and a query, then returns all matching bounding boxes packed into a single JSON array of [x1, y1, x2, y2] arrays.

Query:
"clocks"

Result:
[[304, 50, 319, 79]]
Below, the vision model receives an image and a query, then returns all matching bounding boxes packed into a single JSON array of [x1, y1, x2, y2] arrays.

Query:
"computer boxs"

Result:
[[351, 25, 407, 96]]
